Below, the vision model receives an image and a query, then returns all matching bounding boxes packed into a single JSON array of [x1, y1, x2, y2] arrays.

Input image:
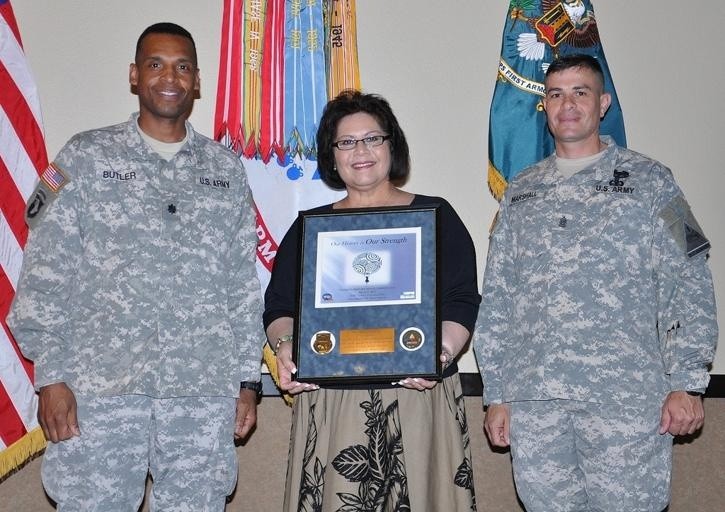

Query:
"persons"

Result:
[[262, 89, 479, 512], [473, 54, 718, 512], [4, 23, 266, 512]]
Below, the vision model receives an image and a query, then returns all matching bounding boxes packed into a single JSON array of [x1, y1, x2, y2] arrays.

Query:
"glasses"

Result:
[[329, 133, 393, 150]]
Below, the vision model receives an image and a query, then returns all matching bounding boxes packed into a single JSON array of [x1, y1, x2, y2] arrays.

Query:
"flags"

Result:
[[0, 1, 51, 479], [488, 0, 627, 198]]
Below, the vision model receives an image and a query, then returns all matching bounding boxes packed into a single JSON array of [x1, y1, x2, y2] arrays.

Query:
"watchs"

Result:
[[240, 380, 262, 398]]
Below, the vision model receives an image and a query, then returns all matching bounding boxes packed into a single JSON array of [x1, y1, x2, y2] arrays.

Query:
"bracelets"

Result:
[[271, 335, 293, 354]]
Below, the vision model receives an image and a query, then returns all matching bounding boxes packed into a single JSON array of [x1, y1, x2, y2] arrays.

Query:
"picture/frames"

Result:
[[290, 206, 445, 386]]
